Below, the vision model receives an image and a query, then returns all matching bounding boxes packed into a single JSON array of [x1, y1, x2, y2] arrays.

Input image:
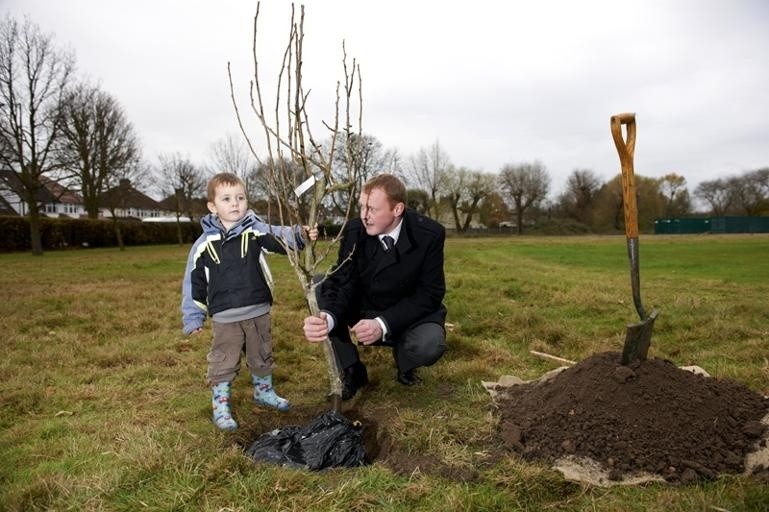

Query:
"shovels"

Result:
[[610, 112, 661, 365]]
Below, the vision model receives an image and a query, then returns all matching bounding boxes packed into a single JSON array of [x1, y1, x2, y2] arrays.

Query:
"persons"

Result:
[[180, 173, 319, 428], [302, 174, 448, 401]]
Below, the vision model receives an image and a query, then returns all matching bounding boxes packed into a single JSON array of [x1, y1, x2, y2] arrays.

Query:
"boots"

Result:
[[252, 374, 291, 411], [212, 381, 238, 431]]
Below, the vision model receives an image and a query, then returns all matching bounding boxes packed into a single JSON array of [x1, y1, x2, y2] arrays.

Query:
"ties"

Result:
[[383, 236, 394, 249]]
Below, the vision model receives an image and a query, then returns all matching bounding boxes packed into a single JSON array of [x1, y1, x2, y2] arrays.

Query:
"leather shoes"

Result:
[[327, 362, 369, 402], [391, 348, 423, 387]]
